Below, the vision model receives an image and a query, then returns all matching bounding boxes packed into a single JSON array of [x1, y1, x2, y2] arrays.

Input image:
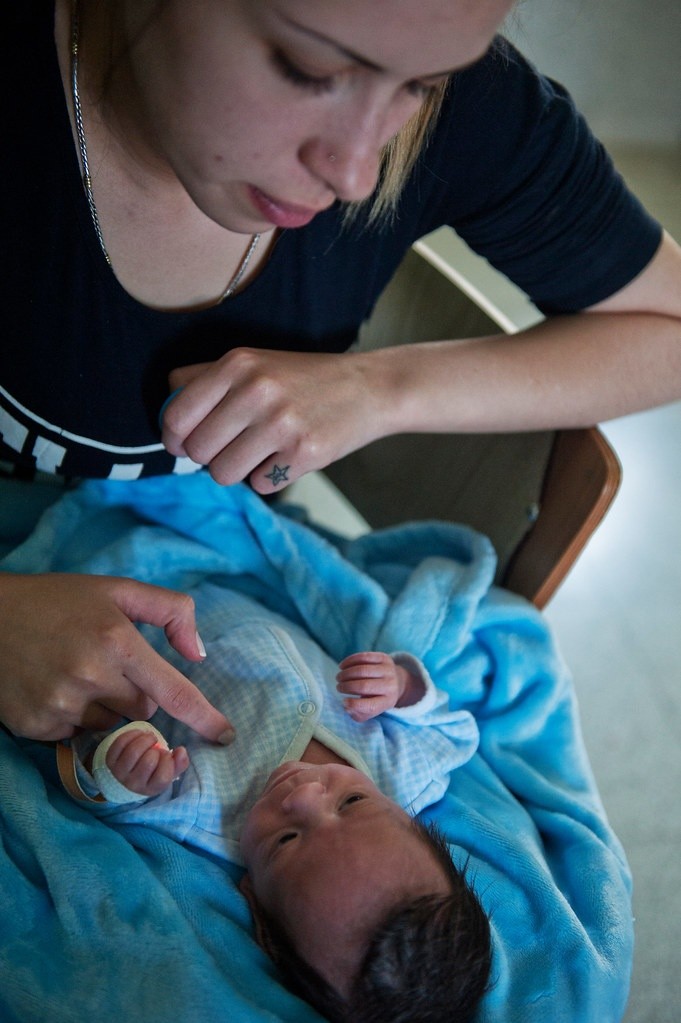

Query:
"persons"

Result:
[[20, 472, 502, 1023], [2, 0, 680, 495]]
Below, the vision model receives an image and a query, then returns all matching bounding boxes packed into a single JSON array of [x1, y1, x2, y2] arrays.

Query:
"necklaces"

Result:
[[61, 22, 268, 306]]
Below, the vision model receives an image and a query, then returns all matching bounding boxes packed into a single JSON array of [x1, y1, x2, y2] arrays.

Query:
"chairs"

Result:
[[279, 225, 622, 612]]
